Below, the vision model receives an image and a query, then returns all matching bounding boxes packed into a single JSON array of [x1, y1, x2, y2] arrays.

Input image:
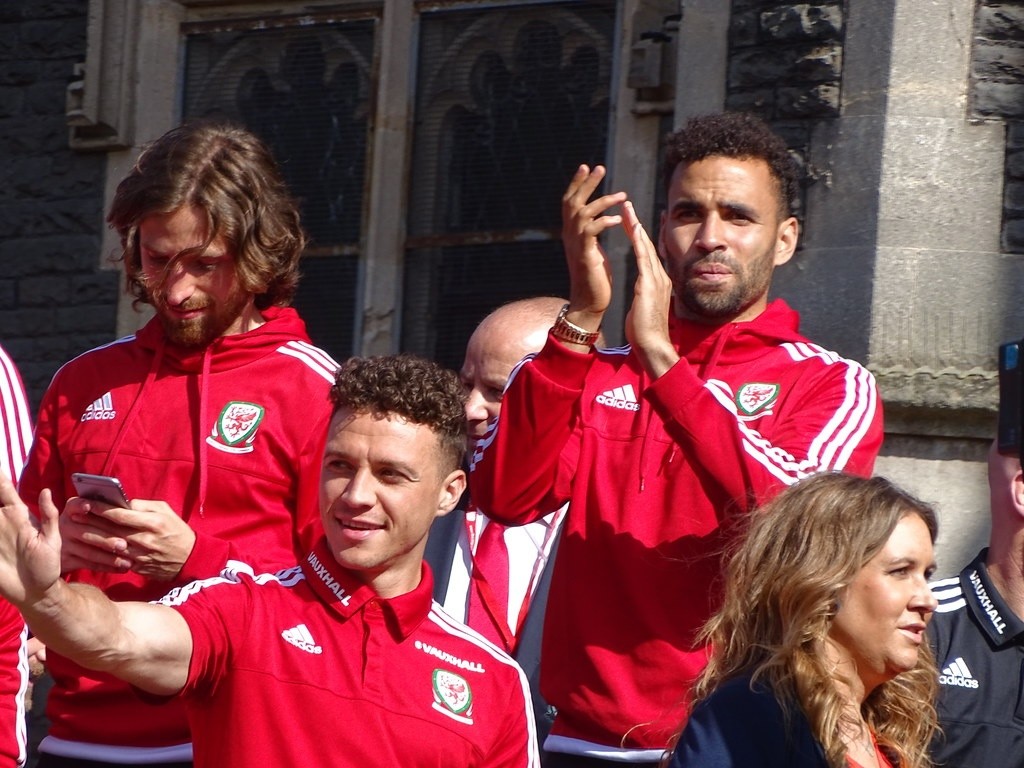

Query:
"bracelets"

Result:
[[553, 304, 602, 345]]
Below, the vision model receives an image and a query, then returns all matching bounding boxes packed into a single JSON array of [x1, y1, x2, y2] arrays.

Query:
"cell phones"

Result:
[[71, 473, 131, 510]]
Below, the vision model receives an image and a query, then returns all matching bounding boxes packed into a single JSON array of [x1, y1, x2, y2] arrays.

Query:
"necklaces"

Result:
[[524, 525, 547, 563], [858, 732, 876, 757]]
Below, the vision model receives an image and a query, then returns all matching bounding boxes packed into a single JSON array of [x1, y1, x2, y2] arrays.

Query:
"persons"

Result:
[[0, 349, 35, 768], [466, 112, 884, 768], [922, 435, 1024, 768], [423, 296, 607, 753], [17, 121, 344, 768], [666, 474, 943, 768], [0, 355, 541, 768]]
